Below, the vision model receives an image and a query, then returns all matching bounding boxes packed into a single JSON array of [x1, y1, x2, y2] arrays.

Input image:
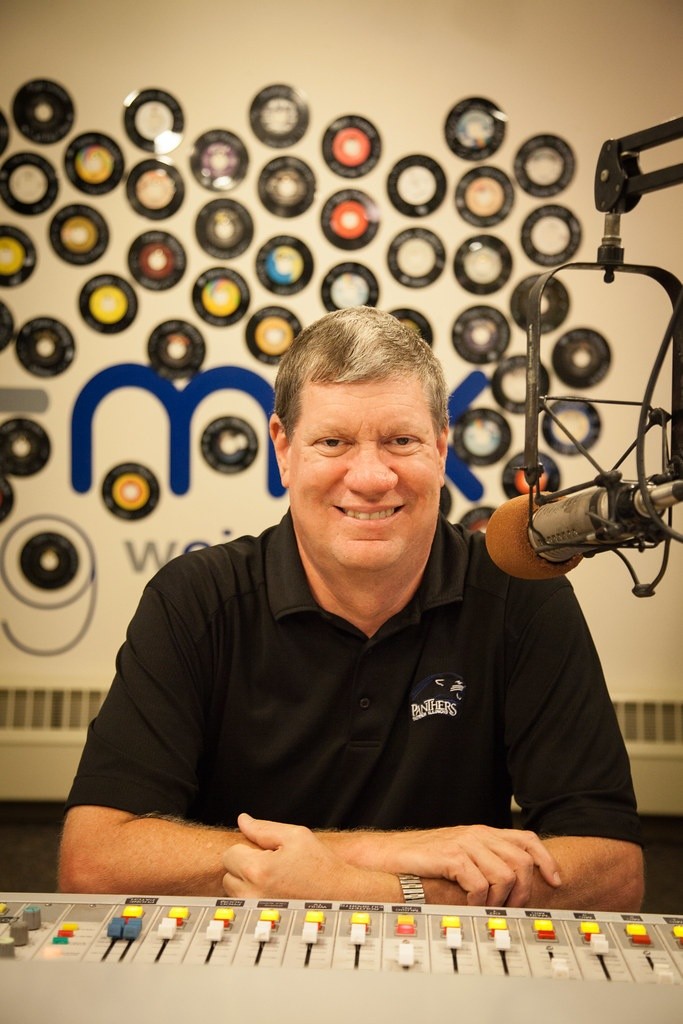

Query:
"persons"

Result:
[[58, 307, 645, 911]]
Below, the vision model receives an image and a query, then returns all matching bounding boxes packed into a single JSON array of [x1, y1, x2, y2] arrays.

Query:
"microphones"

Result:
[[485, 479, 683, 580]]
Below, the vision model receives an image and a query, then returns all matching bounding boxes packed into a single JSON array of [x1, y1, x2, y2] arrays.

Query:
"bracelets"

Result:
[[398, 873, 426, 905]]
